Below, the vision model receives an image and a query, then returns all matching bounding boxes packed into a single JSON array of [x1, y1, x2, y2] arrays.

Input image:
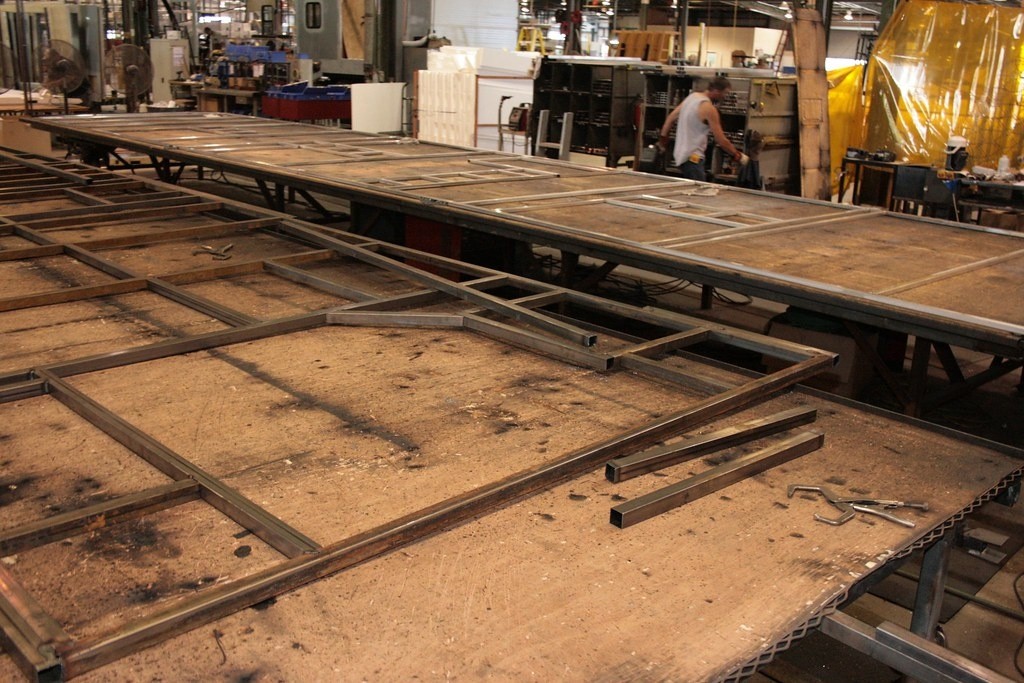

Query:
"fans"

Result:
[[103, 44, 155, 114], [30, 39, 84, 158], [0, 42, 20, 95]]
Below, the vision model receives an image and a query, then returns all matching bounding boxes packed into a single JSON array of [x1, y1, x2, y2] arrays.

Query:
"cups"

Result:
[[1008, 168, 1015, 182]]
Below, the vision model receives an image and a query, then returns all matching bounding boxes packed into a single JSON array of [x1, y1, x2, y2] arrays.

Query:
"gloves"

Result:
[[657, 135, 668, 152], [732, 150, 751, 166]]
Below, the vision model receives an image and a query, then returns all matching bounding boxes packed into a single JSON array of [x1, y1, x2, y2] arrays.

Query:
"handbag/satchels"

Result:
[[735, 159, 761, 189]]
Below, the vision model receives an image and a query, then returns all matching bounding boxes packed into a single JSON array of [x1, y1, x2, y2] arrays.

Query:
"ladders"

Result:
[[607, 30, 684, 65], [515, 26, 546, 55], [769, 29, 790, 71], [534, 110, 574, 161]]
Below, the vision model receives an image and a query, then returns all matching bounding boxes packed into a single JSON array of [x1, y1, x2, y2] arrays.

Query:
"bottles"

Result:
[[972, 166, 994, 177], [998, 155, 1009, 178]]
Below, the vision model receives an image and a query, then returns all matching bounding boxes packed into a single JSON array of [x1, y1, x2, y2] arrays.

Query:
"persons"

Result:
[[654, 77, 750, 181], [204, 26, 221, 57]]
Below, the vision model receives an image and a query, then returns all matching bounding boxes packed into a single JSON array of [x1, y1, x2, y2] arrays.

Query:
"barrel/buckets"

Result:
[[944, 135, 969, 172]]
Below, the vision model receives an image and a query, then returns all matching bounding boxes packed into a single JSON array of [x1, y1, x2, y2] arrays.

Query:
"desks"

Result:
[[838, 158, 1024, 235]]
